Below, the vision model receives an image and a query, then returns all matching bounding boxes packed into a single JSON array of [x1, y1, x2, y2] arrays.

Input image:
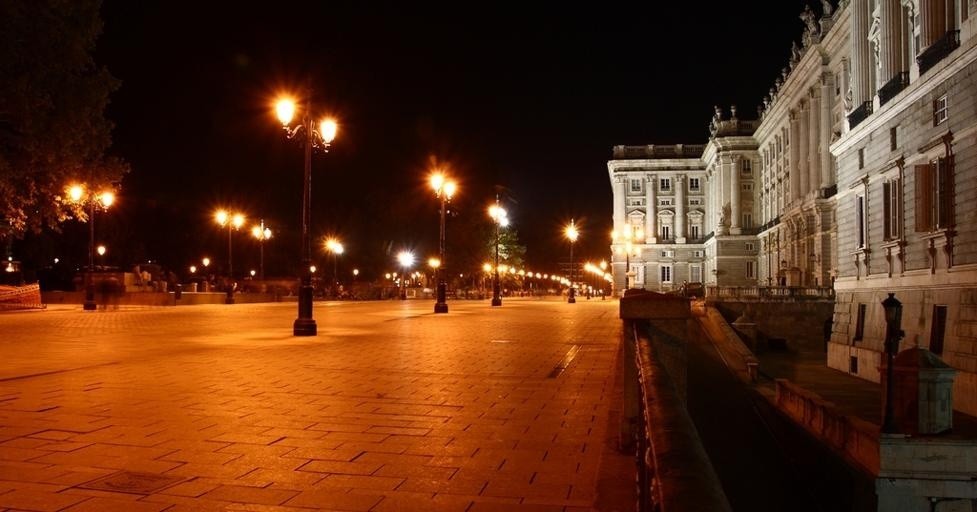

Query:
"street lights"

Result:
[[254, 218, 272, 282], [611, 222, 644, 297], [70, 164, 115, 310], [326, 230, 344, 301], [488, 192, 508, 307], [271, 71, 340, 337], [484, 258, 612, 300], [566, 218, 578, 303], [429, 258, 440, 299], [430, 157, 457, 313], [399, 250, 412, 300], [879, 293, 901, 433], [216, 197, 244, 304]]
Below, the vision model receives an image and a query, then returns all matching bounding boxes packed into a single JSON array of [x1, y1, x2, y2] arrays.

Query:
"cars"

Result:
[[681, 282, 704, 298]]
